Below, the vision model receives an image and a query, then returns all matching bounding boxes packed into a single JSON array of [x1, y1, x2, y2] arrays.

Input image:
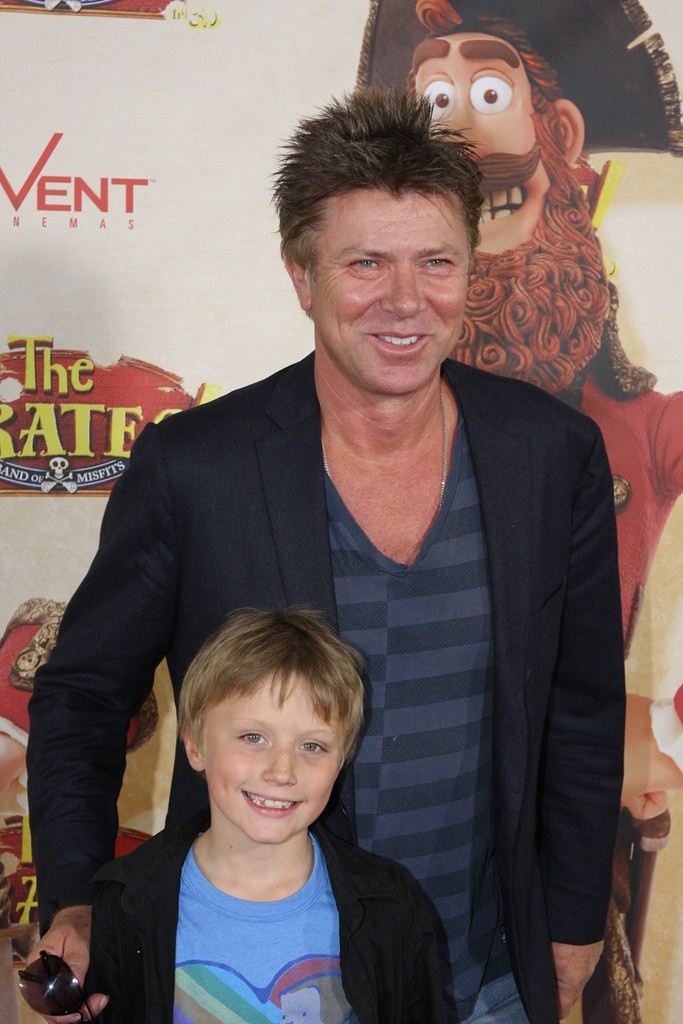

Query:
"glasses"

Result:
[[19, 950, 95, 1024]]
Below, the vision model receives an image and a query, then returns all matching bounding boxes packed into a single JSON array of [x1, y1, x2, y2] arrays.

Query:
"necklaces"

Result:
[[322, 394, 448, 513]]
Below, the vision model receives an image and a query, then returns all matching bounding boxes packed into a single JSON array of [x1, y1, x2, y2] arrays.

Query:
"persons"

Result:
[[25, 95, 626, 1023], [87, 608, 462, 1024]]
